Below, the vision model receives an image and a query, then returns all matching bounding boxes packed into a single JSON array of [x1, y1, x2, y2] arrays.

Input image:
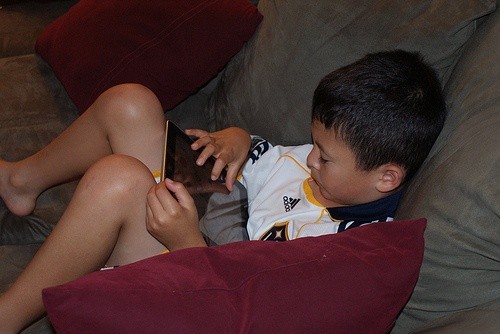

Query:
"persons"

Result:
[[0, 49, 446, 334], [174, 144, 243, 245]]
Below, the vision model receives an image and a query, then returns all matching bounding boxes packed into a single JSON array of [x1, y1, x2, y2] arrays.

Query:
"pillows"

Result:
[[37, 1, 264, 113], [42, 219, 427, 334]]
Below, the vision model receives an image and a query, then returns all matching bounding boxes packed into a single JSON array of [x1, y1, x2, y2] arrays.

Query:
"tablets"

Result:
[[162, 119, 247, 247]]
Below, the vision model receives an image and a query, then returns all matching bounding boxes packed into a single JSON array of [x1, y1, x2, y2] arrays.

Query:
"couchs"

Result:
[[0, 0, 500, 334]]
